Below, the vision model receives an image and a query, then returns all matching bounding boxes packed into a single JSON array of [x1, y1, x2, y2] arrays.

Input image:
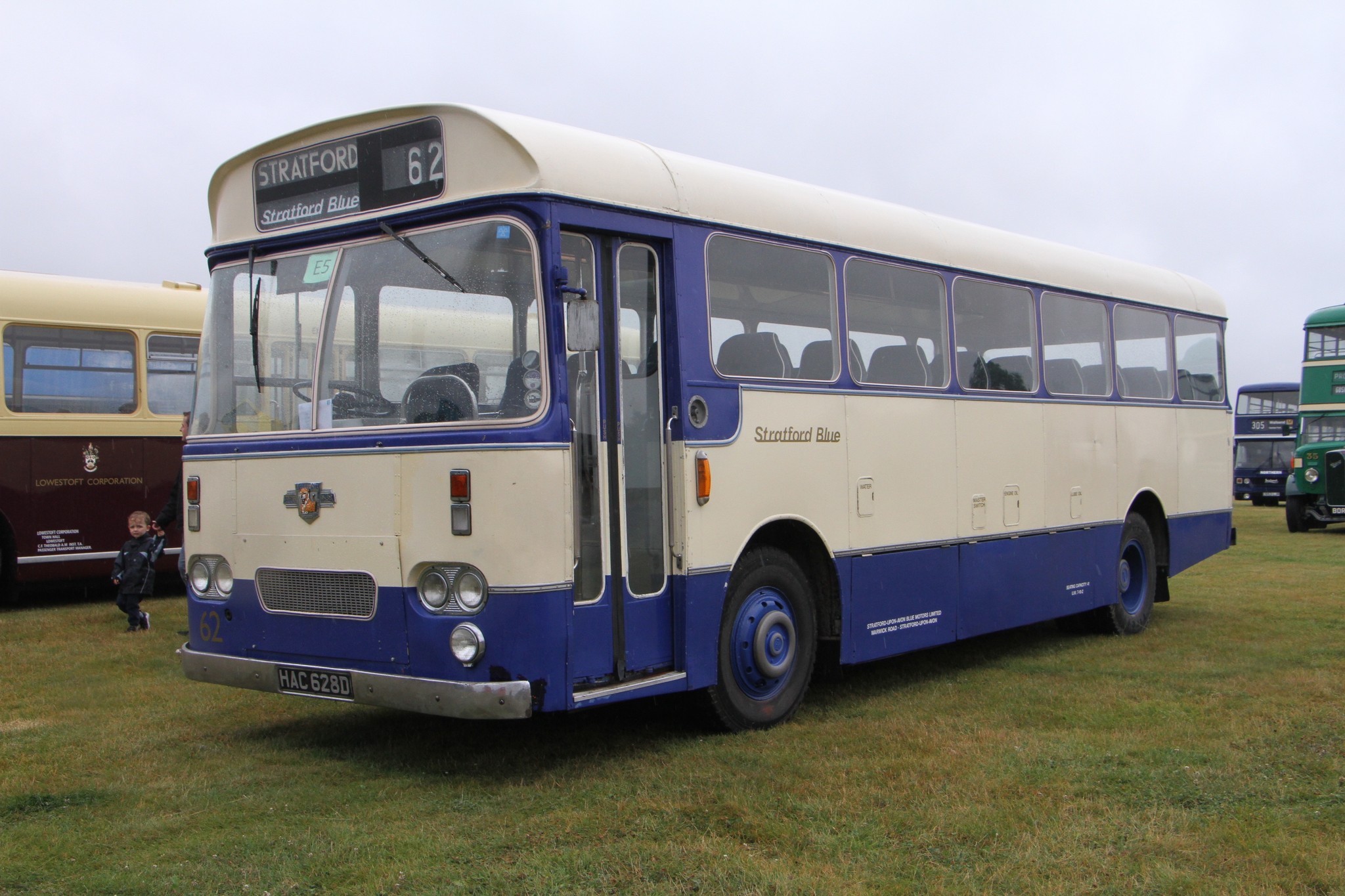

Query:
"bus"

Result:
[[173, 101, 1237, 728], [1282, 303, 1345, 540], [1231, 383, 1300, 510], [0, 268, 536, 582]]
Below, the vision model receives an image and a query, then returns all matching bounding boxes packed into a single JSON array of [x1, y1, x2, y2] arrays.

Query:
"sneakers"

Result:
[[138, 612, 150, 631], [125, 625, 140, 633]]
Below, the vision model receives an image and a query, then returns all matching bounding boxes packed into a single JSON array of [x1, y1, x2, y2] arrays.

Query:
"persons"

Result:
[[111, 511, 168, 633], [152, 411, 194, 637]]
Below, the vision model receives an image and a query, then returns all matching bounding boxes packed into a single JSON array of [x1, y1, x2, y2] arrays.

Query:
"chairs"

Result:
[[408, 362, 480, 421], [715, 333, 1222, 400], [495, 357, 543, 422]]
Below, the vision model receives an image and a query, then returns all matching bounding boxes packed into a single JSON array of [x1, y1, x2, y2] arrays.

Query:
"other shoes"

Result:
[[176, 631, 189, 637]]
